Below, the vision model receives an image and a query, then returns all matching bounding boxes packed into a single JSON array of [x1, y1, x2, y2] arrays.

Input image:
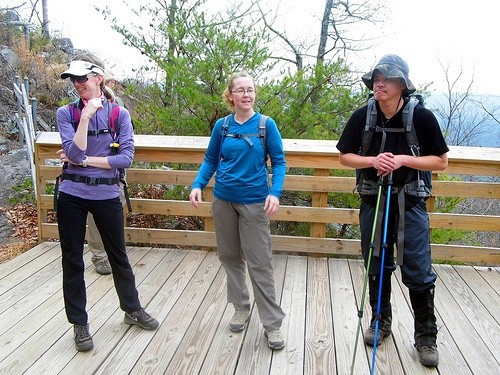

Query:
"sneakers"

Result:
[[73, 323, 93, 351], [364, 325, 389, 345], [124, 308, 158, 330], [417, 344, 440, 367], [229, 310, 251, 332], [95, 260, 111, 274], [264, 328, 285, 350]]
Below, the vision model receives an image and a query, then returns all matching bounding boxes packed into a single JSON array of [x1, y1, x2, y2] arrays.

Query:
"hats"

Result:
[[361, 55, 417, 97], [60, 60, 103, 78]]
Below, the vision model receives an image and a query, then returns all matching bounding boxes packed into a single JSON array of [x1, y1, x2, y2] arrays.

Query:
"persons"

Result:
[[189, 72, 286, 349], [335, 53, 450, 365], [54, 55, 159, 351], [87, 66, 128, 276]]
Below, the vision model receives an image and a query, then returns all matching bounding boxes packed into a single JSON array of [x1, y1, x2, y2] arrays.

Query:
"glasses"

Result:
[[230, 87, 256, 94], [70, 73, 98, 83]]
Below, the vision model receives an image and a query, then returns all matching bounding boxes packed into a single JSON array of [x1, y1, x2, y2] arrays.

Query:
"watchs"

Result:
[[81, 154, 89, 167]]
